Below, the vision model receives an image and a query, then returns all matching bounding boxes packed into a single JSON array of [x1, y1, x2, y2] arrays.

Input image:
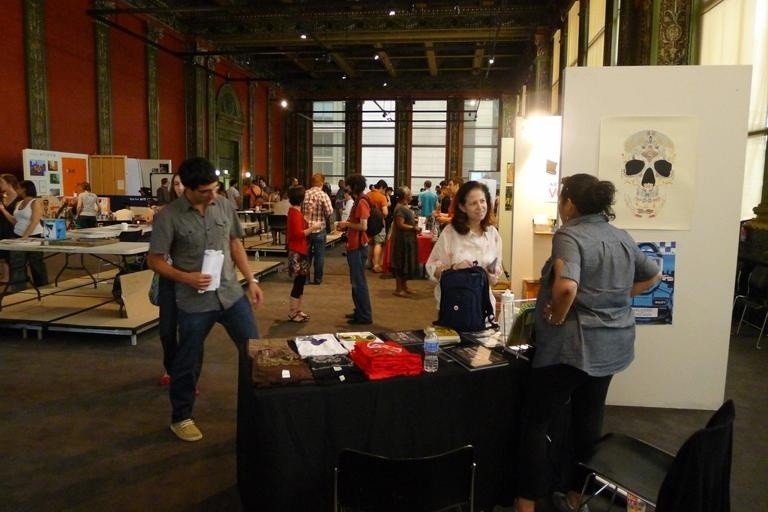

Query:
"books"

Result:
[[198, 249, 224, 293]]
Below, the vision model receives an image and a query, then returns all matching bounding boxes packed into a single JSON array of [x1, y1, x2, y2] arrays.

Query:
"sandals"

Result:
[[297, 310, 310, 320], [286, 314, 305, 322]]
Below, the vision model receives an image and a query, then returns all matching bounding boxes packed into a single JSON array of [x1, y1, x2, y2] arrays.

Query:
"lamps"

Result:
[[370, 51, 379, 60]]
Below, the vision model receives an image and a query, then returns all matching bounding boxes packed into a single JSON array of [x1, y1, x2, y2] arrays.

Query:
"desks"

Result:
[[238, 328, 531, 512], [0, 239, 148, 319], [54, 224, 152, 289], [237, 210, 274, 247], [381, 234, 433, 278]]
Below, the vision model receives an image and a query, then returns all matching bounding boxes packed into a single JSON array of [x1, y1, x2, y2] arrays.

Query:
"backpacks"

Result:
[[366, 204, 384, 238]]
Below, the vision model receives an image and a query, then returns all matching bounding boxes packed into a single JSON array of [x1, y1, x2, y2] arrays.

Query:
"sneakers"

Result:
[[170, 417, 203, 442], [367, 264, 373, 269], [372, 265, 382, 273]]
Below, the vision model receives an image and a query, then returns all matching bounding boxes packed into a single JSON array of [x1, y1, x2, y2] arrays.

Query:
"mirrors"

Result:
[[469, 170, 499, 190]]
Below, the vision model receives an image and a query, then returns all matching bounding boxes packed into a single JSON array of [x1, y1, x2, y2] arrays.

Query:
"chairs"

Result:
[[734, 226, 768, 349], [330, 445, 479, 512], [554, 398, 737, 512], [270, 215, 287, 245]]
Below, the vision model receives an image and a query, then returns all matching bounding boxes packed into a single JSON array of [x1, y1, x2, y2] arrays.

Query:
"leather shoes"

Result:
[[394, 290, 411, 298], [345, 312, 353, 317], [404, 286, 417, 294], [349, 318, 372, 324], [159, 369, 169, 384]]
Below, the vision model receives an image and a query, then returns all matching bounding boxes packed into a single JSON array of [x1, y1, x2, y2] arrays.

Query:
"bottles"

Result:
[[499, 289, 515, 336], [424, 327, 438, 373]]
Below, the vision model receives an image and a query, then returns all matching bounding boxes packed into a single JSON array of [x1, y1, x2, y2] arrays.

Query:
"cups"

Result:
[[255, 205, 259, 212], [418, 217, 425, 228], [121, 223, 128, 231]]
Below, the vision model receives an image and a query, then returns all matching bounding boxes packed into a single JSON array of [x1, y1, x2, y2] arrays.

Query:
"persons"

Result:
[[440, 180, 448, 189], [243, 178, 252, 210], [226, 179, 241, 211], [145, 156, 263, 441], [425, 180, 512, 325], [0, 174, 24, 289], [341, 189, 355, 256], [251, 180, 262, 208], [336, 175, 374, 325], [304, 174, 334, 285], [157, 172, 200, 394], [157, 177, 172, 206], [369, 183, 375, 191], [287, 185, 322, 323], [334, 180, 346, 221], [438, 187, 451, 213], [259, 178, 272, 195], [385, 187, 392, 232], [315, 173, 331, 235], [74, 181, 101, 229], [418, 180, 439, 230], [282, 178, 299, 200], [435, 185, 440, 193], [392, 186, 422, 298], [366, 179, 389, 273], [510, 174, 664, 512], [1, 179, 49, 289], [267, 187, 280, 245], [433, 175, 464, 226]]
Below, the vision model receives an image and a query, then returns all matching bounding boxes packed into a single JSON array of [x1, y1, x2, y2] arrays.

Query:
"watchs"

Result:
[[248, 279, 259, 284]]
